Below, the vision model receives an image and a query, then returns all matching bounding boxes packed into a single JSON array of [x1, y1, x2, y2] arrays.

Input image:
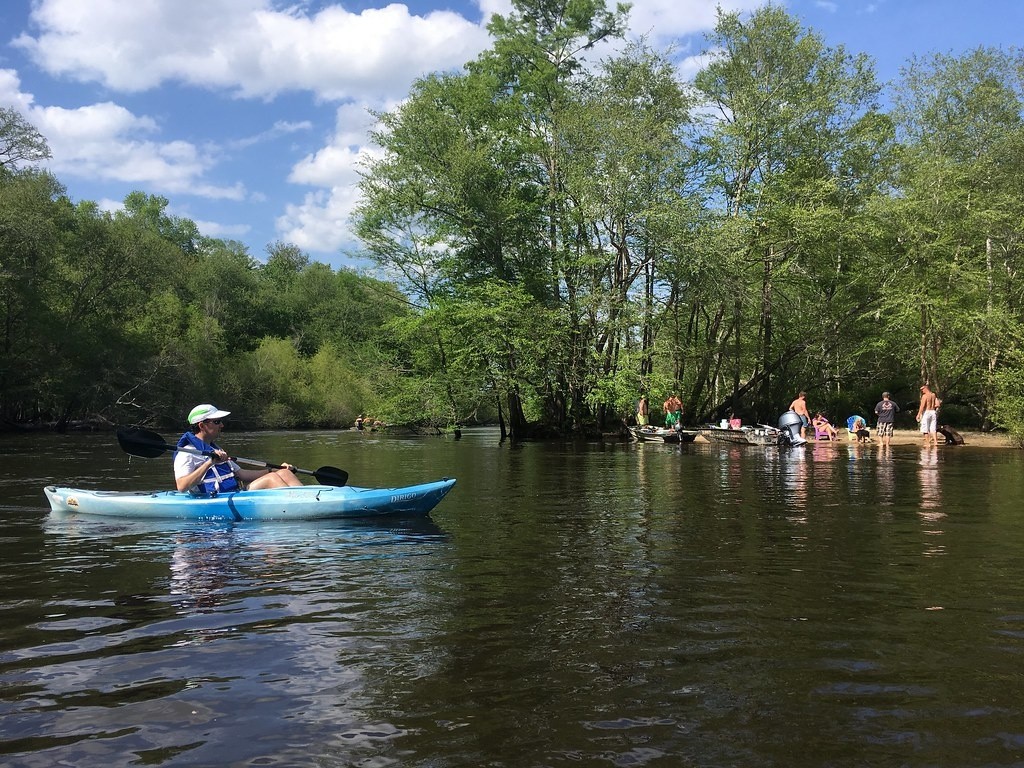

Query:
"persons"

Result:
[[874, 391, 900, 448], [923, 391, 941, 442], [637, 395, 684, 431], [852, 419, 866, 442], [917, 385, 938, 446], [172, 404, 305, 494], [789, 390, 811, 439], [354, 414, 386, 427], [813, 412, 839, 442]]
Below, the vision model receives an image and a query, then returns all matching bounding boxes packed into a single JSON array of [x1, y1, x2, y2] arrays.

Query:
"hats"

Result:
[[188, 404, 231, 424], [204, 419, 224, 425]]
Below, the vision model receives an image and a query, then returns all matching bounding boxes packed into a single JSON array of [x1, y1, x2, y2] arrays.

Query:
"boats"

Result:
[[627, 423, 701, 443], [44, 477, 457, 522], [697, 423, 791, 446]]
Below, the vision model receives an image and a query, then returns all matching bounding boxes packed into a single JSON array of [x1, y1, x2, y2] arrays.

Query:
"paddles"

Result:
[[113, 419, 352, 485]]
